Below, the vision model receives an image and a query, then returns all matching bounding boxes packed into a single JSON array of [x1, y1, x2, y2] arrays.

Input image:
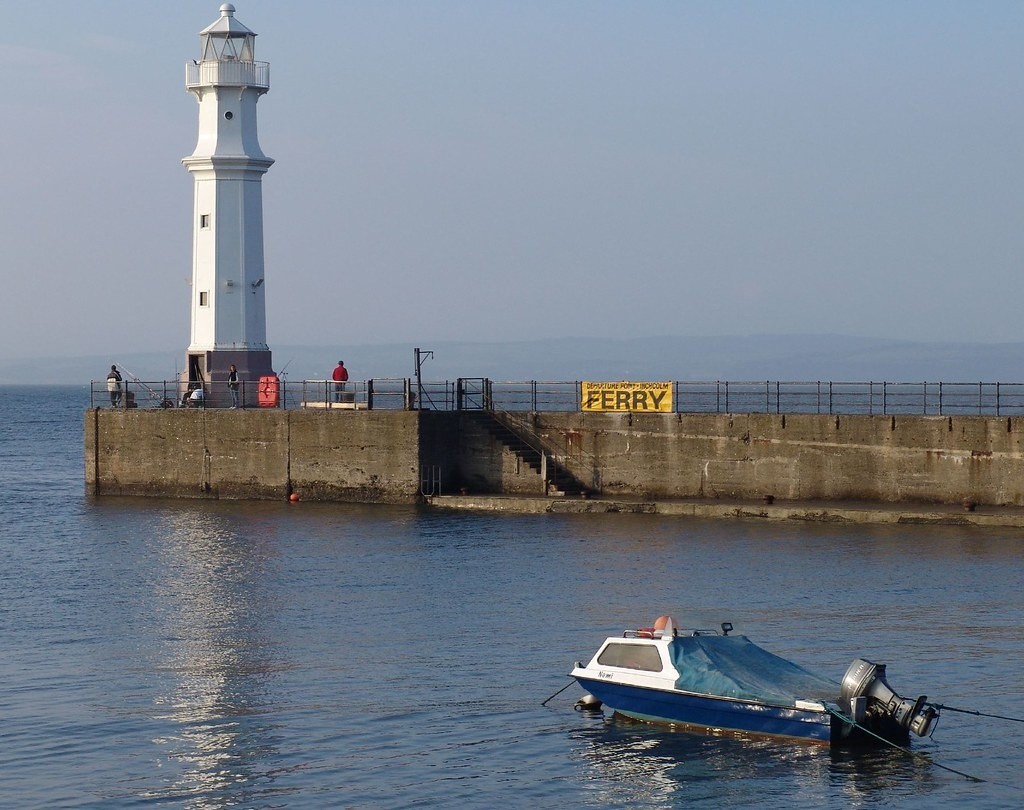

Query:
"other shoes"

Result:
[[232, 407, 237, 409], [229, 407, 232, 409], [113, 405, 115, 408], [119, 405, 121, 408]]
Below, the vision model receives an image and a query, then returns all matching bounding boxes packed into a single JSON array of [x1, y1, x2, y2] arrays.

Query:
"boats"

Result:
[[566, 622, 939, 750]]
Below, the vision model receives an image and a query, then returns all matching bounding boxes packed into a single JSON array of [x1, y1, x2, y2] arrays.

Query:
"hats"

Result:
[[338, 361, 344, 364]]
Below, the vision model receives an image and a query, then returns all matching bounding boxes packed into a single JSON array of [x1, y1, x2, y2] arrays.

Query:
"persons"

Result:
[[183, 387, 203, 408], [106, 365, 122, 408], [332, 361, 348, 402], [228, 365, 239, 409]]
